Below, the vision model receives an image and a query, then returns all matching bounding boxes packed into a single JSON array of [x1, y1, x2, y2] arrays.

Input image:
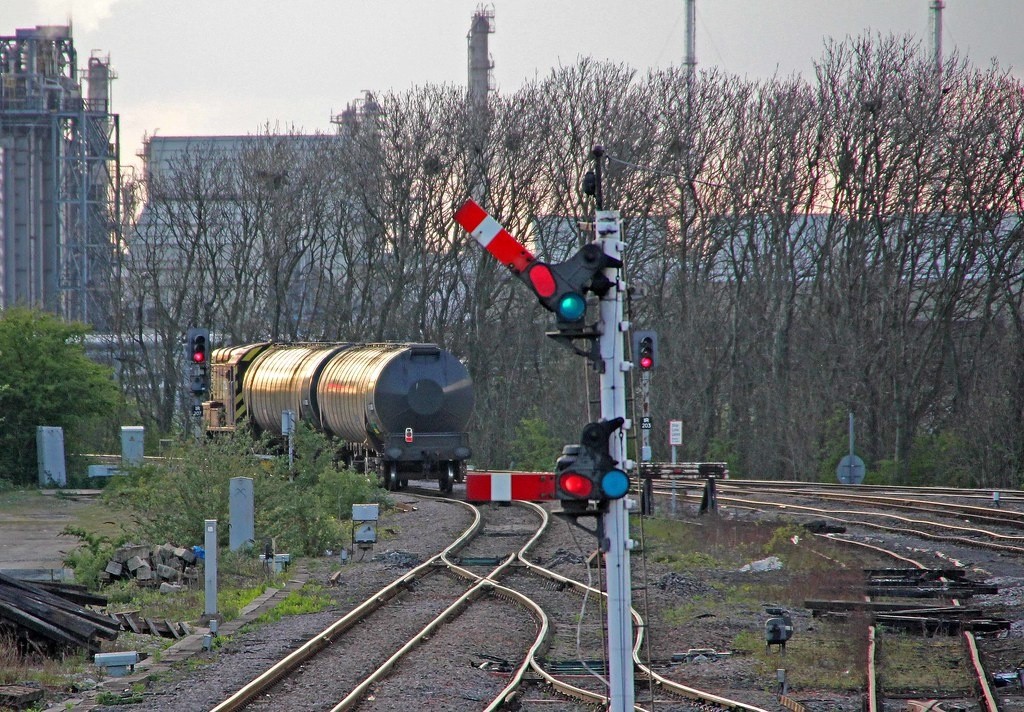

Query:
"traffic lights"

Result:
[[186, 329, 209, 365], [521, 235, 630, 517], [632, 331, 659, 371]]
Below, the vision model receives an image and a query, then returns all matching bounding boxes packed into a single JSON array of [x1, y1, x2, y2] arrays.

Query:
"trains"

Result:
[[203, 340, 473, 493]]
